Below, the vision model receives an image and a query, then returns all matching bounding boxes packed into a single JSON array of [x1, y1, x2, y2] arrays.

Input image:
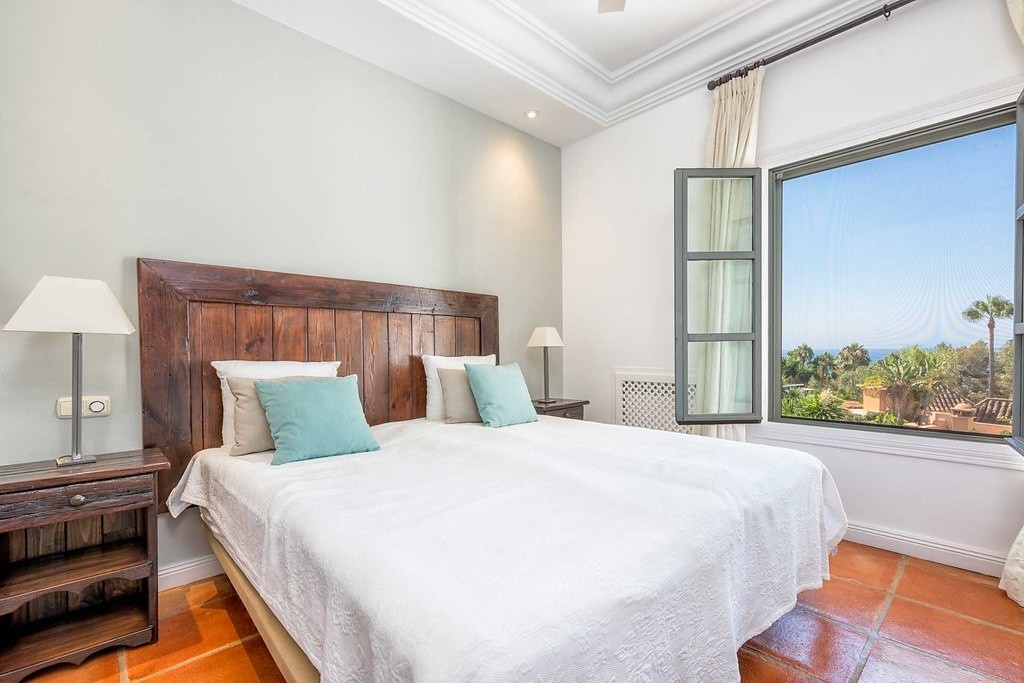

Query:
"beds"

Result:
[[136, 257, 849, 683]]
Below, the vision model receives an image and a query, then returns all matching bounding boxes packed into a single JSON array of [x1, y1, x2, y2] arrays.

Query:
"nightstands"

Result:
[[531, 397, 589, 420], [0, 446, 172, 683]]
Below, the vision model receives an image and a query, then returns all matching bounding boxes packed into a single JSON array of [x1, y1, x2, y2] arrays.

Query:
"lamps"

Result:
[[1, 277, 135, 468], [527, 326, 565, 404]]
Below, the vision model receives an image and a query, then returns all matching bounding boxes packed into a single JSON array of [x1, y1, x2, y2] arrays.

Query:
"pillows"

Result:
[[254, 373, 380, 466], [225, 376, 343, 457], [211, 359, 342, 451], [463, 362, 540, 429], [421, 353, 497, 421], [436, 367, 483, 425]]
